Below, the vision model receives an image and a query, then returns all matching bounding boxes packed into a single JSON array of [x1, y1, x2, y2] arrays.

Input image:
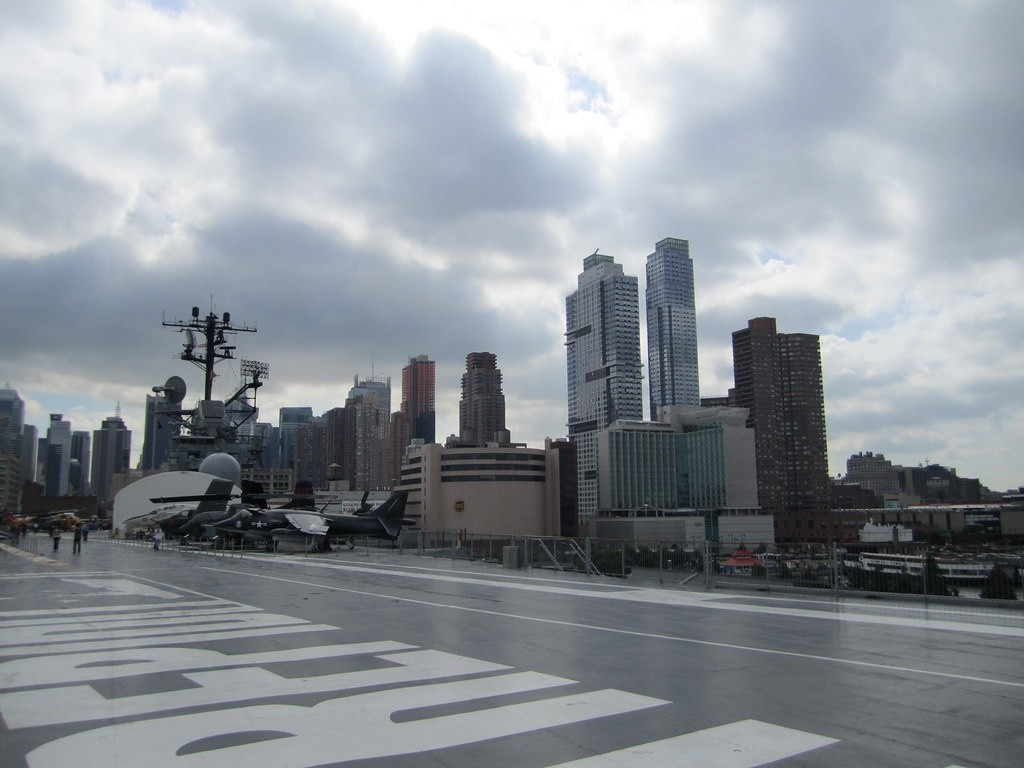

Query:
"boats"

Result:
[[112, 295, 269, 537], [858, 551, 1024, 585]]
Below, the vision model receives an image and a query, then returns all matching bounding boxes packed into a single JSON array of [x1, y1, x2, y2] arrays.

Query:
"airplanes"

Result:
[[5, 508, 110, 536], [122, 478, 417, 553]]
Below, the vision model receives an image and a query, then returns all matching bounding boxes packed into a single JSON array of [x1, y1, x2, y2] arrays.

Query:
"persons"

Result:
[[153, 526, 163, 550], [82, 523, 90, 541], [53, 525, 62, 552], [70, 523, 83, 554]]
